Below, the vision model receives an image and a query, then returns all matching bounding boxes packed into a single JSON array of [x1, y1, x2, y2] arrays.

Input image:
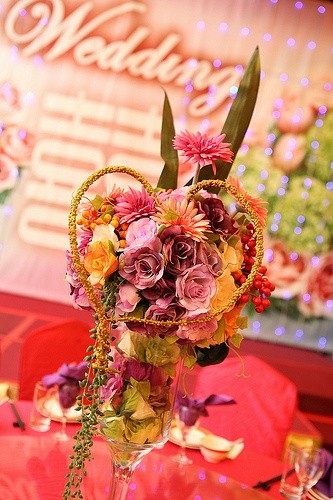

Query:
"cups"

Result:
[[29, 381, 58, 433], [279, 433, 314, 500]]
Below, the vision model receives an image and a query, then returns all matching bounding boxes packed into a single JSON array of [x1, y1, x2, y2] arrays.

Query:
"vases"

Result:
[[89, 322, 186, 500]]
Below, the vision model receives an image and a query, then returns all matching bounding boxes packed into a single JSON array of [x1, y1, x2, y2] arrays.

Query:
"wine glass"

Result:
[[294, 446, 333, 500], [53, 385, 80, 441], [169, 406, 202, 464]]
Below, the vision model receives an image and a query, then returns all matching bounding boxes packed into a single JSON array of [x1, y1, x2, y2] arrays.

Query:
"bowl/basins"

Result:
[[200, 435, 232, 464]]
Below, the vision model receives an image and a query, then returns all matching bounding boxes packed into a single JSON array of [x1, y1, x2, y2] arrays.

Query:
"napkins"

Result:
[[174, 389, 237, 424], [42, 360, 88, 405], [307, 440, 333, 497]]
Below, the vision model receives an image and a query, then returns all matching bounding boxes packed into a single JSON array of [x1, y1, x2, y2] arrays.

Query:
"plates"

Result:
[[163, 426, 207, 450], [45, 398, 82, 423]]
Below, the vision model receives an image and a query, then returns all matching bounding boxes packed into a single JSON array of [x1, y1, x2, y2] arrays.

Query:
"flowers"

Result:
[[39, 41, 273, 500]]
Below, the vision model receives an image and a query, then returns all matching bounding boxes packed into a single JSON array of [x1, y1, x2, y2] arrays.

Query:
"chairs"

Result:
[[195, 355, 297, 454], [18, 318, 96, 402]]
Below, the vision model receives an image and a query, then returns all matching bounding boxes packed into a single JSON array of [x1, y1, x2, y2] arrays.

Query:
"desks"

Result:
[[0, 399, 311, 500]]
